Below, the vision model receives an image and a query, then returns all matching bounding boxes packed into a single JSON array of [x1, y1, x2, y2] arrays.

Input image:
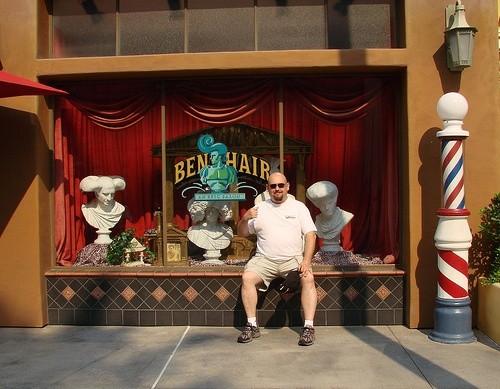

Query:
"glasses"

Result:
[[269, 183, 285, 188]]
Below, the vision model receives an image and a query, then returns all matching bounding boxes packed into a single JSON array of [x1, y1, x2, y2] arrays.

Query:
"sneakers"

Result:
[[298, 324, 316, 346], [237, 322, 261, 343]]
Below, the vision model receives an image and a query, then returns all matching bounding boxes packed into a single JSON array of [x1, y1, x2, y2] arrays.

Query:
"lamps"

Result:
[[444, 0, 478, 72]]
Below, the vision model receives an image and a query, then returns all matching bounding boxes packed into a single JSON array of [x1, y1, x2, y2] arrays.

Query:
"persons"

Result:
[[79, 175, 126, 230], [186, 197, 233, 250], [237, 172, 318, 346], [306, 180, 354, 240]]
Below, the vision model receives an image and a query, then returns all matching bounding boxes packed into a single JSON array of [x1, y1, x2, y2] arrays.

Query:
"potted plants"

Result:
[[477, 192, 500, 344]]
